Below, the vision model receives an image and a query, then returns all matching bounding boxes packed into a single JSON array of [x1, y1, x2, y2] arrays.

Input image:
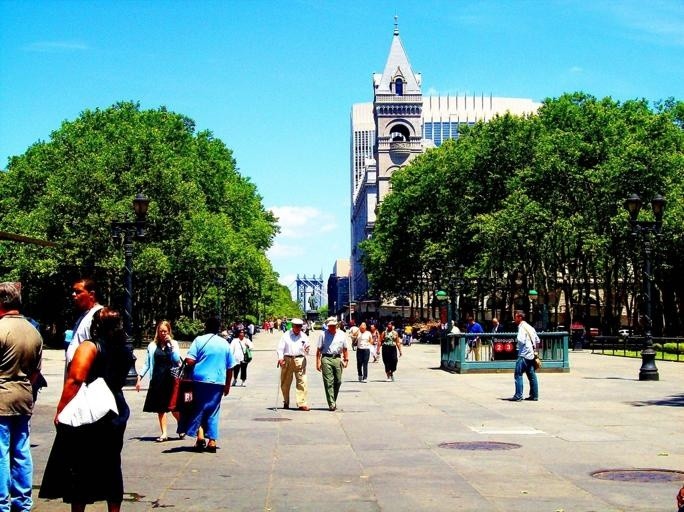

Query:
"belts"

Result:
[[323, 353, 340, 358], [286, 355, 302, 357]]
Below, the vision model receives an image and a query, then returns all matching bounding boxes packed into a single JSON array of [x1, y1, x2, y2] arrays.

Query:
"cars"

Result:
[[590, 328, 598, 335], [619, 329, 635, 337]]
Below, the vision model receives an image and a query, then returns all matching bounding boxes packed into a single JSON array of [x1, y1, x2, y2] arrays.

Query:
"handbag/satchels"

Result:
[[534, 357, 541, 371], [57, 339, 119, 427], [244, 347, 252, 362], [169, 360, 195, 411]]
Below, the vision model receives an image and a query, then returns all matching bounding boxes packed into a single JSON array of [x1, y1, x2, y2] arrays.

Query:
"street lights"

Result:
[[234, 282, 273, 327], [112, 191, 153, 388], [620, 190, 669, 381], [208, 263, 226, 325]]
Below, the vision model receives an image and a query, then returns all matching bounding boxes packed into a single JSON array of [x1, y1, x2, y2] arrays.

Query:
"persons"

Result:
[[316, 316, 349, 413], [63, 276, 108, 389], [219, 316, 507, 362], [176, 318, 234, 454], [277, 318, 310, 411], [349, 322, 377, 383], [509, 308, 541, 402], [37, 307, 130, 512], [229, 329, 254, 386], [134, 321, 188, 442], [1, 280, 43, 511], [308, 293, 317, 309], [377, 320, 402, 382]]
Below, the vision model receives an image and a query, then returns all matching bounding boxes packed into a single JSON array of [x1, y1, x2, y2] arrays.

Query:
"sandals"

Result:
[[195, 439, 216, 453]]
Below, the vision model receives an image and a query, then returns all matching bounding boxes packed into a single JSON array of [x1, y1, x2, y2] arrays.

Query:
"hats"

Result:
[[325, 316, 338, 326], [291, 318, 303, 325]]
